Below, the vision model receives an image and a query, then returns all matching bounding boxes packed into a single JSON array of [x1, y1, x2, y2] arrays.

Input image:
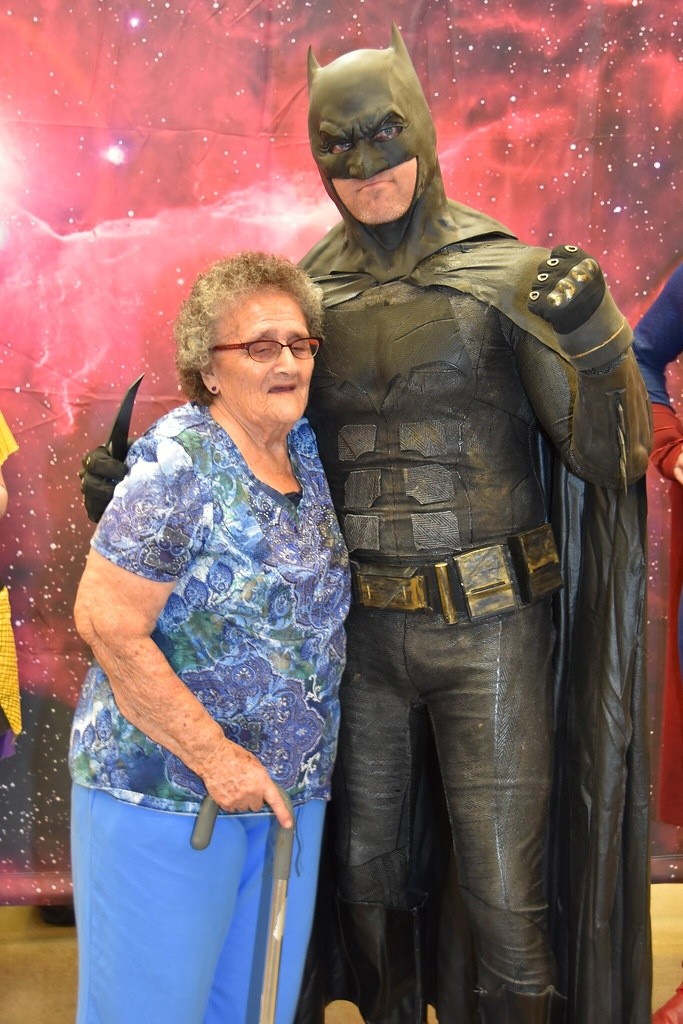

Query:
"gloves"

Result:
[[528, 245, 634, 373], [79, 445, 130, 522]]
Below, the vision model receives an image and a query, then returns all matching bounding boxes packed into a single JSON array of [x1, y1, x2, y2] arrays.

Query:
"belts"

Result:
[[347, 524, 565, 625]]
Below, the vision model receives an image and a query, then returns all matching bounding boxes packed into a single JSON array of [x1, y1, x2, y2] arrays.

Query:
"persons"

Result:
[[67, 251, 355, 1024], [86, 38, 646, 1021], [635, 266, 680, 1021]]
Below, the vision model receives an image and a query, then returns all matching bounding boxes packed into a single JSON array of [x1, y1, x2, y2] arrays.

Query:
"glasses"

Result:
[[207, 337, 320, 363]]
